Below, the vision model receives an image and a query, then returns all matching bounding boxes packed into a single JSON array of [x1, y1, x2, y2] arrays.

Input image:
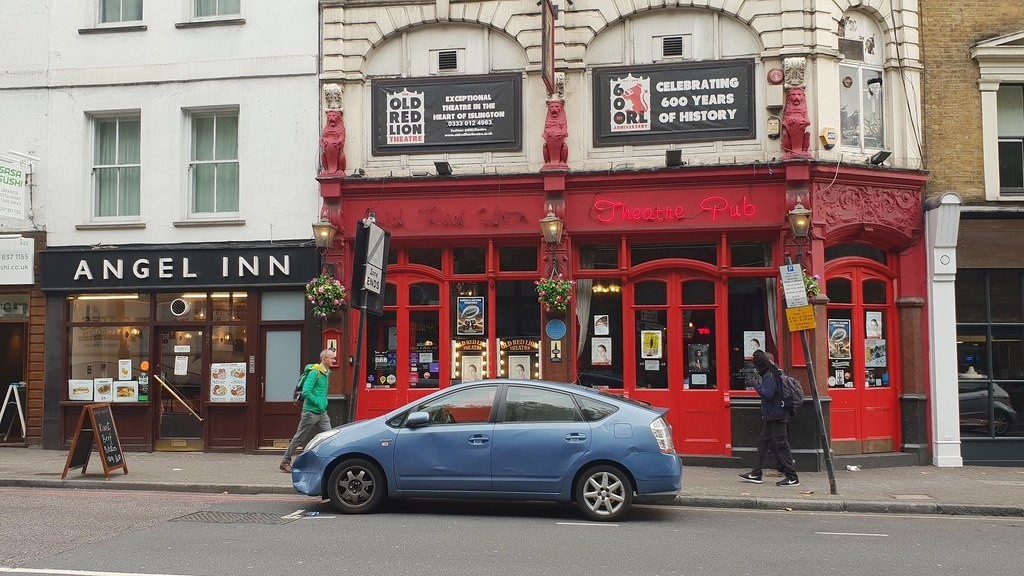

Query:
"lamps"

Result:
[[435, 161, 453, 175], [538, 205, 564, 275], [785, 196, 812, 264], [871, 150, 892, 166], [311, 211, 336, 276], [666, 148, 683, 166]]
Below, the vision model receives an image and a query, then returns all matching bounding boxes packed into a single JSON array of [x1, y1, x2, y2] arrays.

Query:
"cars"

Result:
[[958, 373, 1018, 438], [290, 378, 684, 523]]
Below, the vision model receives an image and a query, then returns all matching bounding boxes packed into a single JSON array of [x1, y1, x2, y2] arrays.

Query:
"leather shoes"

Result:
[[280, 461, 292, 473]]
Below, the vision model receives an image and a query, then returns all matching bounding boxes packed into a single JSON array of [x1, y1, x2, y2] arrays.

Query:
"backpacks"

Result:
[[293, 367, 320, 404], [765, 368, 804, 409]]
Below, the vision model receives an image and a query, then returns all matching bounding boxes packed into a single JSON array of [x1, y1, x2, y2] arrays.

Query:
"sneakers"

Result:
[[776, 477, 800, 486], [739, 471, 762, 483]]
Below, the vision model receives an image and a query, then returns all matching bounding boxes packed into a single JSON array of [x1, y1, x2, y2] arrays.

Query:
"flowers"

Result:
[[779, 269, 819, 305], [534, 267, 576, 314], [305, 273, 347, 322]]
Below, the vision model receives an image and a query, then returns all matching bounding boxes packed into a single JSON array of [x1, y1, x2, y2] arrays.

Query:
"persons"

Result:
[[870, 319, 880, 335], [515, 364, 527, 380], [750, 338, 760, 355], [465, 365, 481, 381], [694, 350, 704, 371], [596, 345, 610, 361], [739, 348, 802, 487], [279, 349, 336, 473]]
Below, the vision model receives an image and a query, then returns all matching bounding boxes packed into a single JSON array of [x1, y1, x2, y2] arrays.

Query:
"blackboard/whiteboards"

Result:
[[66, 403, 125, 471], [0, 383, 27, 438]]
